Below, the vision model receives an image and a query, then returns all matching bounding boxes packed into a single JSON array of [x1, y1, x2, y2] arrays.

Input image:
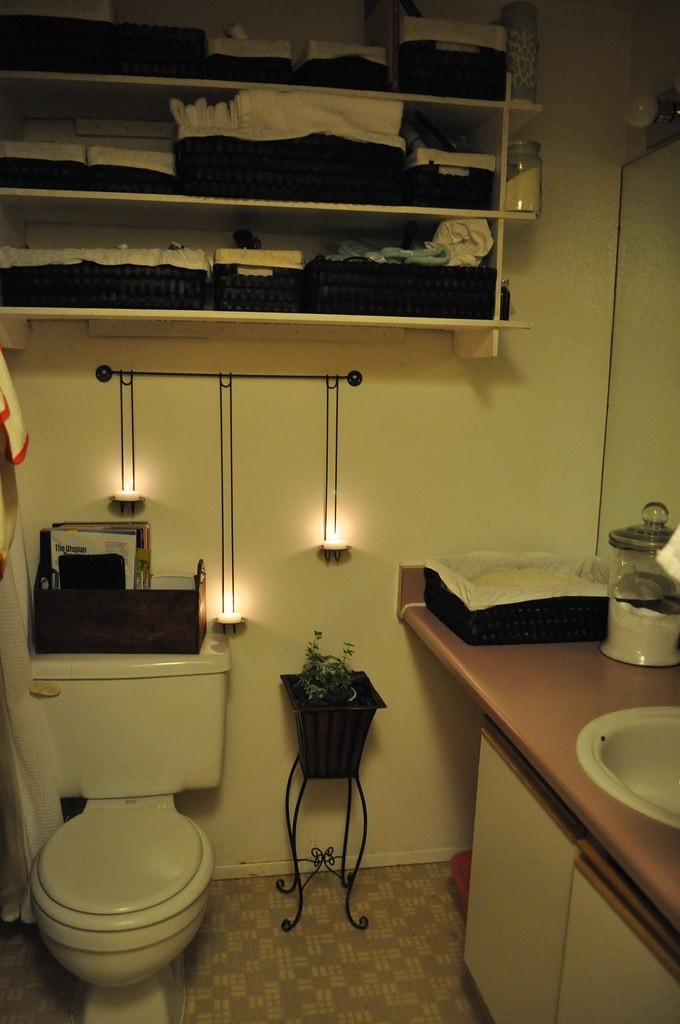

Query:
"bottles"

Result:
[[500, 279, 510, 321]]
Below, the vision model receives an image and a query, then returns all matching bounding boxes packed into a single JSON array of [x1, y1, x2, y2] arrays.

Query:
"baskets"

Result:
[[303, 254, 497, 319], [0, 261, 208, 309], [422, 567, 610, 646], [0, 14, 505, 210], [212, 263, 303, 314]]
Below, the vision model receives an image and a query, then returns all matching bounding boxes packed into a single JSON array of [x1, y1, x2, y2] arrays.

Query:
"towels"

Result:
[[305, 237, 451, 264], [0, 346, 30, 580], [172, 89, 403, 142]]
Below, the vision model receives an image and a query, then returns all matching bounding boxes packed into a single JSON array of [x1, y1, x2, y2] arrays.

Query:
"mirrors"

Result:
[[594, 136, 680, 590]]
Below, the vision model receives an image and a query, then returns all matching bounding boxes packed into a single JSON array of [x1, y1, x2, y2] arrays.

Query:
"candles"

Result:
[[218, 605, 241, 624], [116, 478, 139, 501], [323, 535, 348, 550]]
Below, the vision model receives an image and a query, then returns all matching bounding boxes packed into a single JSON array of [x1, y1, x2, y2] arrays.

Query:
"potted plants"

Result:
[[280, 631, 388, 780]]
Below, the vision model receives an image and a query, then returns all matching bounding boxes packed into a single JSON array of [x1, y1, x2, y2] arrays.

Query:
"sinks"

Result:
[[594, 712, 678, 823]]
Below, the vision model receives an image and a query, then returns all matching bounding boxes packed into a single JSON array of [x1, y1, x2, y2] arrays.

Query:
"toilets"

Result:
[[27, 634, 231, 1021]]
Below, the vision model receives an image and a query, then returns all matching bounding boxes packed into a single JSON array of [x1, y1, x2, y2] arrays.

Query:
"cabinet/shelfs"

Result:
[[462, 712, 680, 1024], [0, 69, 543, 361]]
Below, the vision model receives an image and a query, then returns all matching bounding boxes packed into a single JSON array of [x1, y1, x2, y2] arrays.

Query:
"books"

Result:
[[40, 522, 150, 589]]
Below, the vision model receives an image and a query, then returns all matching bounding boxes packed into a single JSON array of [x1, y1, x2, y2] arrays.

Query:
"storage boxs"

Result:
[[32, 558, 207, 655], [0, 12, 512, 322]]
